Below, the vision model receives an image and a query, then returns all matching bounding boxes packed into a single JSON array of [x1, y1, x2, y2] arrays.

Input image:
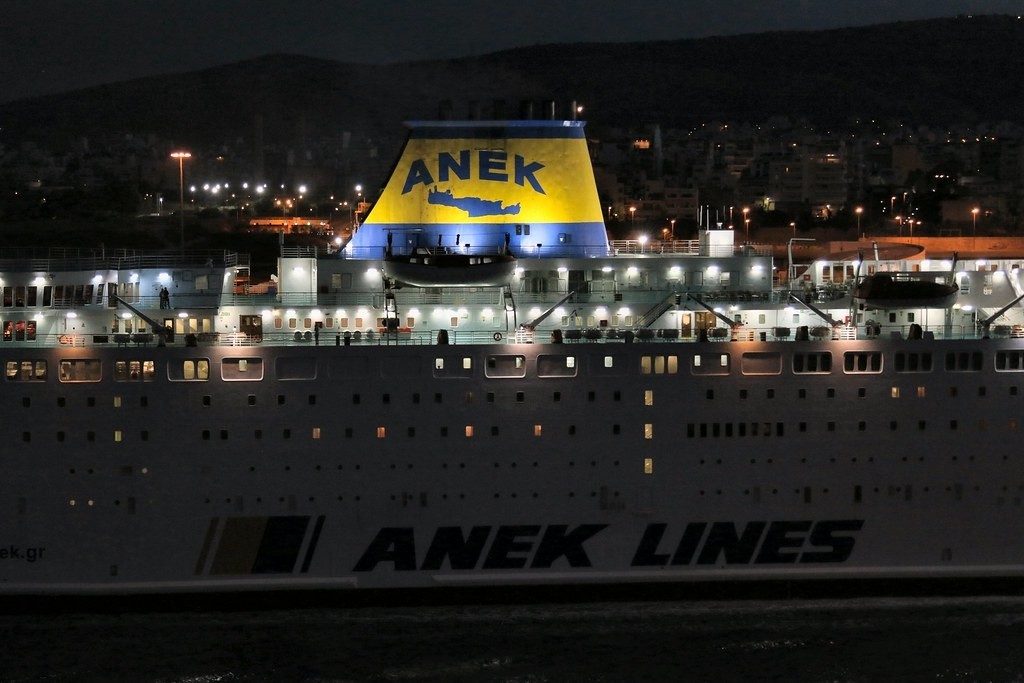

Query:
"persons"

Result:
[[131, 370, 139, 380], [981, 323, 991, 340], [519, 324, 535, 344], [159, 287, 171, 310]]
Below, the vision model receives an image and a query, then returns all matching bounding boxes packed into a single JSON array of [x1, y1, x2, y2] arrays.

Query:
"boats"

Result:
[[0, 120, 1024, 636]]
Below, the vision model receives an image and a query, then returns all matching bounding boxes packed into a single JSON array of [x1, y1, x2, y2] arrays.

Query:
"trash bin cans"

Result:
[[697, 329, 708, 341], [760, 332, 766, 341], [551, 330, 563, 343], [797, 326, 808, 340], [439, 330, 448, 344], [185, 334, 197, 346], [344, 337, 350, 345]]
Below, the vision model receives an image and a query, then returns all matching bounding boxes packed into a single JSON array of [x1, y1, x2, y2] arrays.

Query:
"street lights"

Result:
[[671, 220, 676, 241], [790, 222, 796, 244], [903, 191, 908, 206], [743, 208, 749, 232], [630, 206, 635, 229], [909, 219, 913, 237], [896, 216, 902, 238], [745, 219, 750, 245], [890, 196, 896, 218], [663, 228, 668, 241], [970, 207, 980, 259], [170, 151, 192, 264], [855, 207, 864, 240]]
